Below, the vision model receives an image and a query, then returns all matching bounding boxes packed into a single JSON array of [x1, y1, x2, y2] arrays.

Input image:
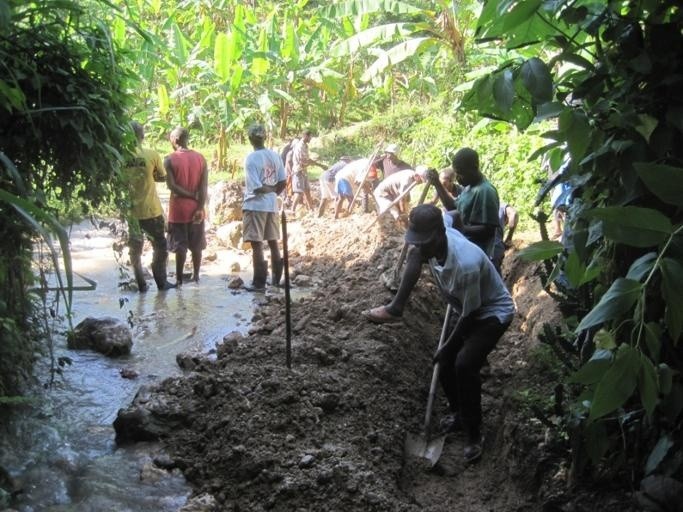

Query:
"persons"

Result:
[[163, 128, 206, 287], [113, 121, 175, 291], [241, 125, 285, 291], [404, 204, 514, 464], [361, 148, 498, 323], [541, 133, 575, 240], [281, 130, 517, 273]]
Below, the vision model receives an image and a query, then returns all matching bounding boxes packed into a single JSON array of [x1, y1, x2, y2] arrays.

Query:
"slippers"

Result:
[[464, 445, 481, 462], [362, 306, 402, 323]]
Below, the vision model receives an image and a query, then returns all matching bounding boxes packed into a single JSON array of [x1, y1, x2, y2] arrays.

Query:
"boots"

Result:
[[266, 258, 283, 285], [245, 261, 268, 291], [151, 262, 177, 290], [134, 267, 150, 292]]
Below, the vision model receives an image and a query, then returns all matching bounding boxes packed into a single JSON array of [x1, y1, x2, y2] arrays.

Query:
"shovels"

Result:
[[401, 303, 453, 471]]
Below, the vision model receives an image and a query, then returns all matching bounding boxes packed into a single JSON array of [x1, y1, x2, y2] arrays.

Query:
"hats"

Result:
[[384, 145, 398, 155], [448, 147, 480, 168], [405, 205, 443, 244]]
